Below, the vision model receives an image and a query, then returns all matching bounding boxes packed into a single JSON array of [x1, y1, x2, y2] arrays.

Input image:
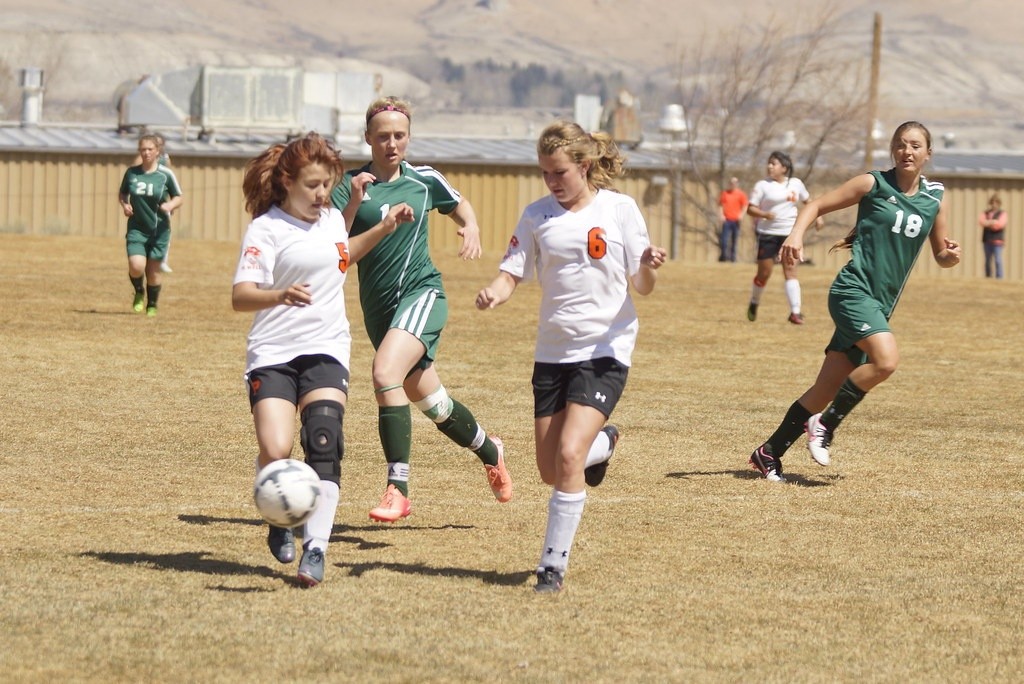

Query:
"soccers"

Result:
[[251, 457, 323, 530]]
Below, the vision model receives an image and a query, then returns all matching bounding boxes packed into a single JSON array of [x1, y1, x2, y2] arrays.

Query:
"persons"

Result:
[[749, 120, 962, 482], [752, 216, 781, 263], [476, 123, 666, 594], [979, 194, 1008, 279], [330, 96, 512, 523], [232, 131, 415, 586], [718, 177, 748, 262], [747, 151, 824, 326], [134, 133, 173, 273], [119, 135, 185, 316]]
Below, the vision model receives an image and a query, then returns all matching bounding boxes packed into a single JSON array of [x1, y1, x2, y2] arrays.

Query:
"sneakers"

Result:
[[133, 290, 145, 311], [147, 302, 156, 316], [482, 435, 513, 504], [749, 446, 788, 483], [369, 484, 412, 520], [585, 426, 619, 487], [748, 302, 756, 321], [804, 413, 834, 466], [534, 572, 564, 597], [268, 526, 295, 563], [789, 313, 804, 325], [299, 539, 324, 585]]
